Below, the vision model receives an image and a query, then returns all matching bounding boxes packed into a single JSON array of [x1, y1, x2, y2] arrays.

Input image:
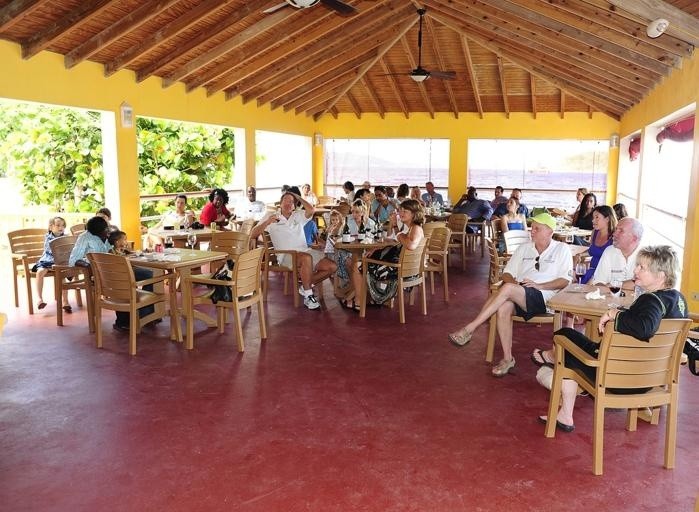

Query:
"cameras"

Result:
[[192, 222, 204, 229]]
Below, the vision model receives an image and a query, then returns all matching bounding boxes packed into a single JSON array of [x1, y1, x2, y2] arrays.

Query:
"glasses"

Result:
[[535, 256, 539, 272]]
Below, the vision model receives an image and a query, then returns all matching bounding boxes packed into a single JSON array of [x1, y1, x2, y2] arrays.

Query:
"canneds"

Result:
[[155, 244, 162, 252]]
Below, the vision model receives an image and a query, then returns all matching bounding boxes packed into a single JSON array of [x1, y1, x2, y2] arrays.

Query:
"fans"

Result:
[[262, 0, 359, 19], [374, 8, 459, 83]]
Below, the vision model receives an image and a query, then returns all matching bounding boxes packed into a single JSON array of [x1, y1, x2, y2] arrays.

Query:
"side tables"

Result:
[[149, 227, 227, 291], [311, 203, 342, 227], [424, 209, 452, 223], [334, 238, 401, 304], [548, 281, 645, 366], [124, 247, 229, 341], [550, 226, 595, 268]]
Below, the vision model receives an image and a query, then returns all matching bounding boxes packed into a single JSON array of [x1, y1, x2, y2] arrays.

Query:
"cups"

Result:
[[164, 237, 173, 248], [144, 253, 154, 262], [128, 241, 135, 250], [210, 222, 216, 232], [198, 224, 205, 229], [191, 221, 199, 230], [566, 234, 574, 246], [426, 208, 432, 215], [155, 244, 162, 252]]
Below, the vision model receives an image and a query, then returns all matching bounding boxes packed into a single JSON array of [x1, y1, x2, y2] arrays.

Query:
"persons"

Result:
[[32, 209, 159, 333], [454, 185, 530, 256], [200, 189, 234, 227], [146, 194, 194, 250], [565, 188, 643, 330], [531, 245, 688, 433], [325, 181, 444, 311], [449, 214, 574, 375], [234, 185, 265, 221], [250, 184, 338, 310]]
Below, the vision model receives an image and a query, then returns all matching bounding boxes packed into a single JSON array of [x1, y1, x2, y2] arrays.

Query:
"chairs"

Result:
[[543, 318, 694, 476], [485, 218, 563, 362], [359, 199, 491, 324], [8, 219, 323, 356]]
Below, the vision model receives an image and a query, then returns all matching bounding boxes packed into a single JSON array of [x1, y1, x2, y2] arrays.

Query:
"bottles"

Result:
[[435, 201, 440, 214], [373, 213, 380, 230], [357, 216, 366, 242], [563, 209, 567, 219], [247, 209, 254, 218], [342, 217, 351, 244], [430, 204, 434, 215], [440, 203, 445, 215], [366, 228, 388, 243]]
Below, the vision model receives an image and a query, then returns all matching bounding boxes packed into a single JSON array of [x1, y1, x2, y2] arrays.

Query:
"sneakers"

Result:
[[493, 357, 516, 377], [299, 285, 320, 310], [448, 327, 472, 346]]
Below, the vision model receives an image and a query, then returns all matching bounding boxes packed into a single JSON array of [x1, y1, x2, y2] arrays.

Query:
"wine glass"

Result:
[[608, 280, 623, 309], [188, 233, 197, 256], [575, 263, 587, 290]]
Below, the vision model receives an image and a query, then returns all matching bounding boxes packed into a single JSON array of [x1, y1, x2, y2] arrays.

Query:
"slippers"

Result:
[[531, 348, 554, 368], [537, 416, 575, 432]]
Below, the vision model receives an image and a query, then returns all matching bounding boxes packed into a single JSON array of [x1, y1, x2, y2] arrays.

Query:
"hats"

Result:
[[527, 213, 557, 231]]
